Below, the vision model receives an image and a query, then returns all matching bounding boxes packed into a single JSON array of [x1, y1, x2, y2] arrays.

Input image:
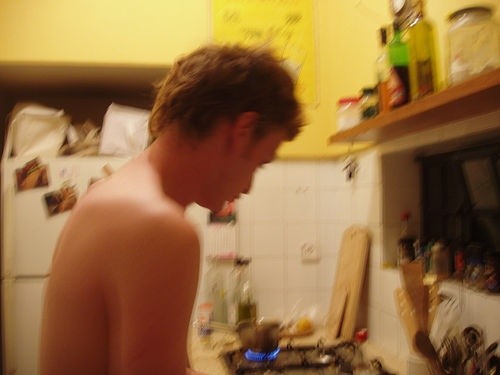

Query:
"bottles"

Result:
[[408, 11, 440, 101], [428, 243, 449, 281], [359, 89, 379, 122], [376, 28, 390, 114], [384, 20, 411, 111], [397, 211, 417, 264]]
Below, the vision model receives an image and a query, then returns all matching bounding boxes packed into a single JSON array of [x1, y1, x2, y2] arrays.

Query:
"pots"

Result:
[[235, 319, 280, 353]]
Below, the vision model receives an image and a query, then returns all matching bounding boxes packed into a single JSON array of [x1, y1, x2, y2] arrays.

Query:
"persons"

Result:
[[39, 42, 302, 375]]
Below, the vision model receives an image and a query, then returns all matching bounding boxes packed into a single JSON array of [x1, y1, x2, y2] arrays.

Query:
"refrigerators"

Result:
[[0, 157, 208, 374]]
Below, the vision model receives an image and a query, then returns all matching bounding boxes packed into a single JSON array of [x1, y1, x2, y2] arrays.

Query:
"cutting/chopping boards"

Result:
[[325, 224, 370, 342]]
